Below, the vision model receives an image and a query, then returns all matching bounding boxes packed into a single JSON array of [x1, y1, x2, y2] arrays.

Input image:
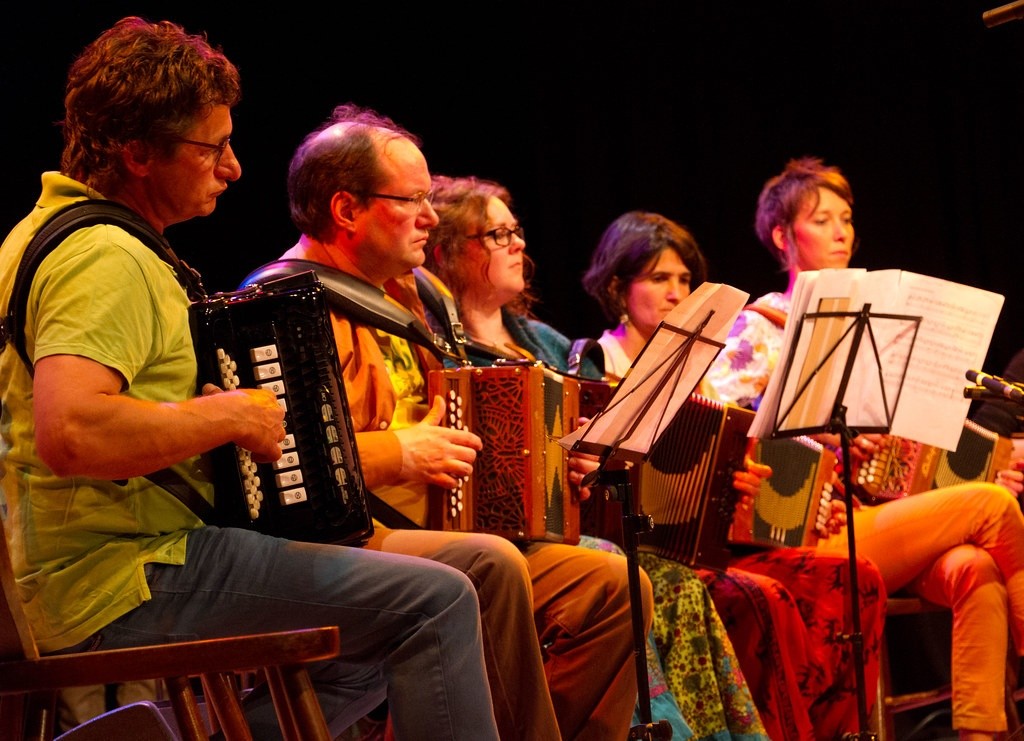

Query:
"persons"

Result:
[[707, 157, 1023, 741], [585, 213, 883, 741], [243, 102, 654, 740], [0, 16, 561, 740], [422, 175, 772, 740]]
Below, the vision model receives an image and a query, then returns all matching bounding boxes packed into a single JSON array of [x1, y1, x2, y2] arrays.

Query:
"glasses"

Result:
[[354, 185, 434, 215], [163, 138, 230, 165], [444, 224, 525, 247]]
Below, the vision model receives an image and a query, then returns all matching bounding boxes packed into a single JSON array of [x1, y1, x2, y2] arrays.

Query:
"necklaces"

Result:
[[464, 330, 505, 347]]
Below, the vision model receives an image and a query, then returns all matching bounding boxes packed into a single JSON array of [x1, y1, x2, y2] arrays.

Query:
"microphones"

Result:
[[963, 370, 1024, 407]]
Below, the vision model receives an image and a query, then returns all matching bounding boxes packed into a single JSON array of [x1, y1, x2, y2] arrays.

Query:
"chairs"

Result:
[[0, 503, 1023, 741]]
